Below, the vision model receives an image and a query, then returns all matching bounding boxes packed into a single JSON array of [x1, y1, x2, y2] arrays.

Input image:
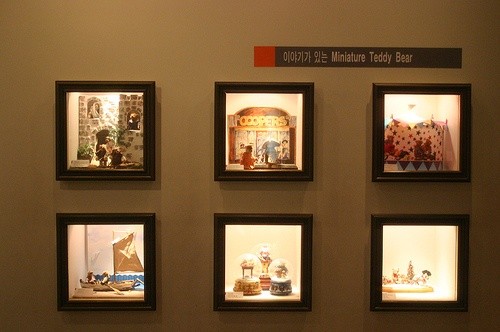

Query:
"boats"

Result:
[[80, 278, 113, 288]]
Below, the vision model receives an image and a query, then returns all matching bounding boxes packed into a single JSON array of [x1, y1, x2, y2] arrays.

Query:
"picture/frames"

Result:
[[372, 82, 472, 183], [213, 213, 312, 312], [213, 82, 314, 182], [55, 213, 157, 311], [370, 214, 469, 311], [55, 81, 156, 181]]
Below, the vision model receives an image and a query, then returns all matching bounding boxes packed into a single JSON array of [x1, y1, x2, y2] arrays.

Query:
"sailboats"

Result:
[[91, 230, 144, 291]]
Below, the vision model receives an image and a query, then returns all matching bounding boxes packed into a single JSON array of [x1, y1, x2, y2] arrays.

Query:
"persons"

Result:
[[241, 145, 255, 170], [95, 145, 123, 168]]
